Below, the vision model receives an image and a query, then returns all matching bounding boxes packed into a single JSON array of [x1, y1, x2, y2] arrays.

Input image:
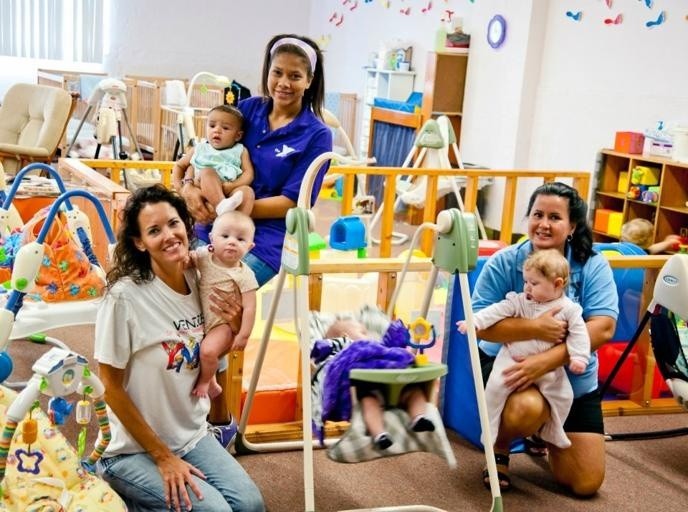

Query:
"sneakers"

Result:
[[206, 413, 237, 449]]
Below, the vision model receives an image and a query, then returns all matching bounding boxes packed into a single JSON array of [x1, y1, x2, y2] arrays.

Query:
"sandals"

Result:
[[482, 454, 510, 490], [524, 435, 547, 456]]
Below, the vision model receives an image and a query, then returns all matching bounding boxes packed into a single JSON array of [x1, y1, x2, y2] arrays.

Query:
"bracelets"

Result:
[[184, 176, 194, 186]]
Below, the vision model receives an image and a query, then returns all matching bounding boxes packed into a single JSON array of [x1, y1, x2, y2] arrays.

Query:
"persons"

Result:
[[187, 33, 333, 289], [455, 248, 588, 450], [325, 318, 434, 450], [467, 183, 620, 497], [179, 212, 260, 398], [172, 104, 256, 217], [96, 181, 267, 512], [608, 218, 681, 255]]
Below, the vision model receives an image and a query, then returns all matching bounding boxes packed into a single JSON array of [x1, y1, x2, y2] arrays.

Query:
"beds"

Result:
[[34, 68, 358, 164]]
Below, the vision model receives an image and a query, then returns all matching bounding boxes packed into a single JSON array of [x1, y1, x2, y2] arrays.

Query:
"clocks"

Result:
[[487, 14, 506, 49]]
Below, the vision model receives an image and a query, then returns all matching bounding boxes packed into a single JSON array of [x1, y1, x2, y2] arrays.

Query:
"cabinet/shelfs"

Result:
[[586, 149, 688, 253], [358, 67, 415, 165], [421, 49, 468, 151]]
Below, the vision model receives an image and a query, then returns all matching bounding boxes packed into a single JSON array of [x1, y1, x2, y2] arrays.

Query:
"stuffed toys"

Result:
[[625, 165, 661, 206], [672, 234, 688, 254]]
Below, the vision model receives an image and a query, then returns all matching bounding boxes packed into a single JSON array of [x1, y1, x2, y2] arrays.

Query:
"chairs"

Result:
[[0, 81, 81, 178]]
